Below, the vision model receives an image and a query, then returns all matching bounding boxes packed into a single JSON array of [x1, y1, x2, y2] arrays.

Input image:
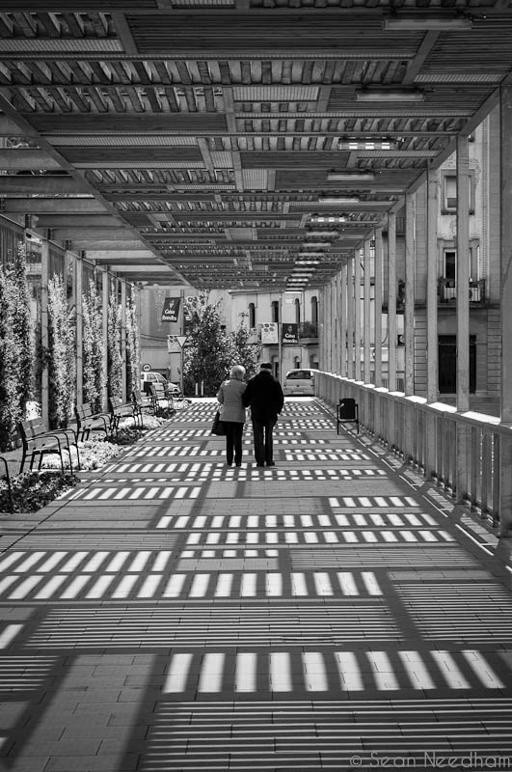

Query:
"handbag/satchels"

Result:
[[211, 411, 226, 436]]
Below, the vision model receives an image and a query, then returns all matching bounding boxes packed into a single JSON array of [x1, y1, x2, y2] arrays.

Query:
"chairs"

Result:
[[336, 396, 359, 435]]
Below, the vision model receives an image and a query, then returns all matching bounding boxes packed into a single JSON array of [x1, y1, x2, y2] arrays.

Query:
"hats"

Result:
[[261, 363, 272, 369]]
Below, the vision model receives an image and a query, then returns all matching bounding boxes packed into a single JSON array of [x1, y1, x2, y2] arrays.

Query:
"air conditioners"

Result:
[[469, 287, 482, 303]]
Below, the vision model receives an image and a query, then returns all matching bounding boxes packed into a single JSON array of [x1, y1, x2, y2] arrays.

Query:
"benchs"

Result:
[[0, 382, 173, 514]]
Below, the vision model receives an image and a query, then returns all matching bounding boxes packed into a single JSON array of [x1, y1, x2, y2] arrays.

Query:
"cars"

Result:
[[284, 367, 319, 396], [140, 372, 179, 396]]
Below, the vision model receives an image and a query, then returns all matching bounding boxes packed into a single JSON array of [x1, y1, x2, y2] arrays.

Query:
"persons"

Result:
[[216, 365, 250, 466], [242, 362, 284, 466], [285, 324, 295, 339]]
